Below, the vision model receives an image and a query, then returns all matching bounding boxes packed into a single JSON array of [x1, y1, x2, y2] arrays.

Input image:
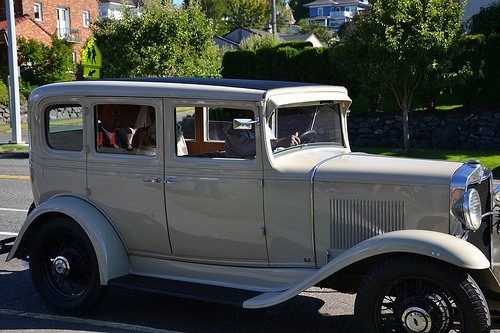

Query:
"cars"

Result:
[[5, 76, 499, 333]]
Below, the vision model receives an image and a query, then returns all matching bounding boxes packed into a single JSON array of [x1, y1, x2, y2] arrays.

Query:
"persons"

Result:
[[225, 109, 301, 158], [94, 104, 156, 156]]
